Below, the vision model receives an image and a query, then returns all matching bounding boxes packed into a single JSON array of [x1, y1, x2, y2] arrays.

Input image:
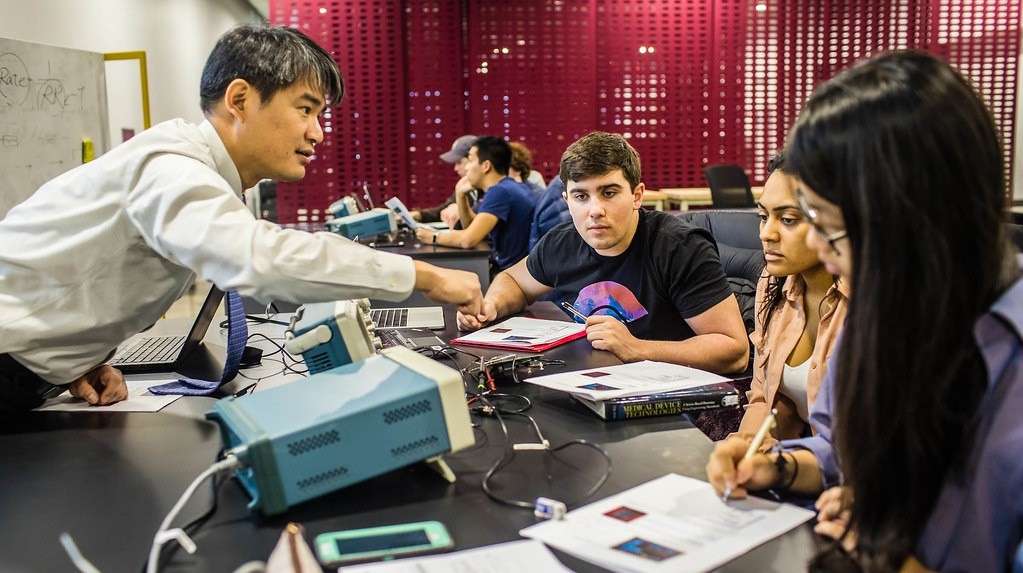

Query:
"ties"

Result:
[[149, 284, 249, 396]]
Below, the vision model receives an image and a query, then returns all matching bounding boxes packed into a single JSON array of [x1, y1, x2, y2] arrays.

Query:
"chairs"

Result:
[[672, 208, 765, 380], [705, 164, 750, 207]]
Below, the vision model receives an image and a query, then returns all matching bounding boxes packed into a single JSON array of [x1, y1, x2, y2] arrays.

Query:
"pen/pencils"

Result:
[[560, 302, 589, 327], [721, 408, 779, 500]]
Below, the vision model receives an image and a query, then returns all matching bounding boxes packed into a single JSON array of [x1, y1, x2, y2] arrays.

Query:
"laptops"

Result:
[[372, 327, 457, 359], [364, 185, 375, 210], [366, 306, 446, 332], [103, 285, 226, 369]]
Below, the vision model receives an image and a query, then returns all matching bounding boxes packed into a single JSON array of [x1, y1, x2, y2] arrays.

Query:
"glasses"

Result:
[[795, 189, 849, 255]]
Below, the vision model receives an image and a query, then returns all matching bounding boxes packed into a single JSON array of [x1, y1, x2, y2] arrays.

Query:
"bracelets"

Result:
[[433, 232, 439, 245], [774, 451, 799, 495]]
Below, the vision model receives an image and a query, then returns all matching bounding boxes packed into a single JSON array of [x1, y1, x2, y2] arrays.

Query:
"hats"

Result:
[[439, 135, 478, 163]]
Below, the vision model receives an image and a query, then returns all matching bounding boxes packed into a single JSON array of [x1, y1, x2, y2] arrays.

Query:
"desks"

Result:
[[642, 190, 668, 210], [0, 300, 836, 573], [661, 187, 764, 211], [279, 222, 494, 305]]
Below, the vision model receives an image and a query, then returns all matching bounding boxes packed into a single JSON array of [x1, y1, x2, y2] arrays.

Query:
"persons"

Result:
[[707, 49, 1022, 573], [0, 26, 487, 419], [395, 135, 547, 284], [529, 173, 576, 303], [456, 132, 750, 373], [728, 154, 849, 450]]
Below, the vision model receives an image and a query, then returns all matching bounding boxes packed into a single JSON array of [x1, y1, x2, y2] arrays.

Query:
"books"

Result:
[[570, 382, 740, 420]]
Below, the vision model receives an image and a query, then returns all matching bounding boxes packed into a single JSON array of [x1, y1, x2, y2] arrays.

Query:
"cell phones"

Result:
[[314, 519, 455, 569], [369, 241, 404, 247]]
